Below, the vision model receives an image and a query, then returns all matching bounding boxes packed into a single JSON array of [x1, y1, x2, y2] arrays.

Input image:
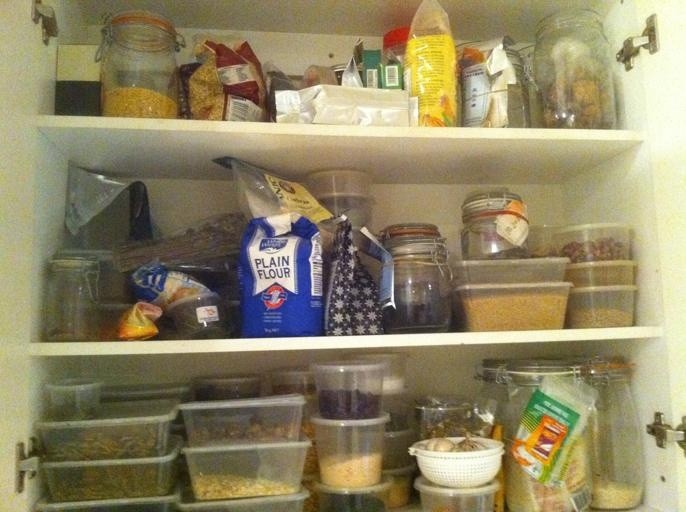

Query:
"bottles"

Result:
[[382, 25, 410, 89], [44, 252, 102, 340], [474, 357, 644, 509], [533, 9, 619, 128], [455, 41, 538, 131], [379, 222, 454, 332], [99, 10, 179, 118], [460, 190, 529, 257]]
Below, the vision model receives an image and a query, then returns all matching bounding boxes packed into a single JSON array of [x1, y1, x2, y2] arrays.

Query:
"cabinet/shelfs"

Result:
[[0, 2, 686, 512]]
